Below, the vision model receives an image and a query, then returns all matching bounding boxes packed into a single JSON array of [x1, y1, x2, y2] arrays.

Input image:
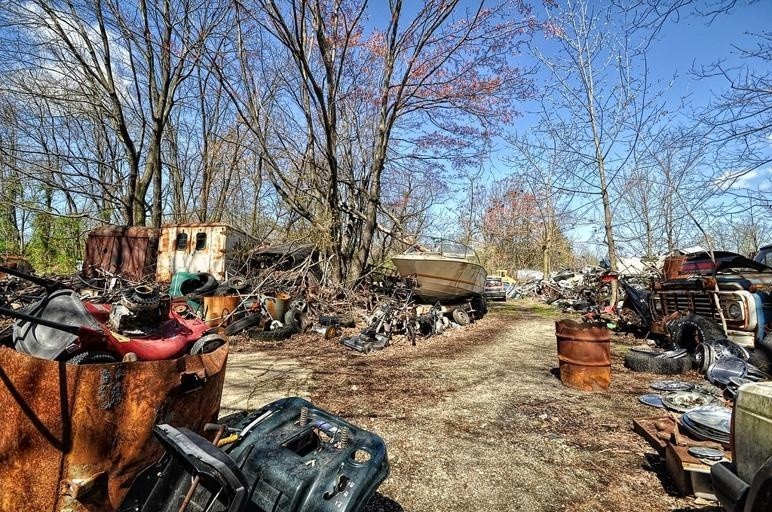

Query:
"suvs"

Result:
[[485, 277, 506, 300]]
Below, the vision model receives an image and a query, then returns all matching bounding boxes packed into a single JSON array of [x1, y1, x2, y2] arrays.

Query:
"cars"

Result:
[[650, 245, 772, 346]]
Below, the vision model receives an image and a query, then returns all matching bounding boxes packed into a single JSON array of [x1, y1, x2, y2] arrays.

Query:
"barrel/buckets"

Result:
[[202, 295, 237, 333], [262, 291, 292, 323], [554, 321, 612, 390], [236, 293, 259, 319], [0, 332, 231, 512]]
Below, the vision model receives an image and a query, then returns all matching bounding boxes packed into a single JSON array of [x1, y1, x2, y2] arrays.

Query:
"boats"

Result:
[[390, 255, 490, 303]]
